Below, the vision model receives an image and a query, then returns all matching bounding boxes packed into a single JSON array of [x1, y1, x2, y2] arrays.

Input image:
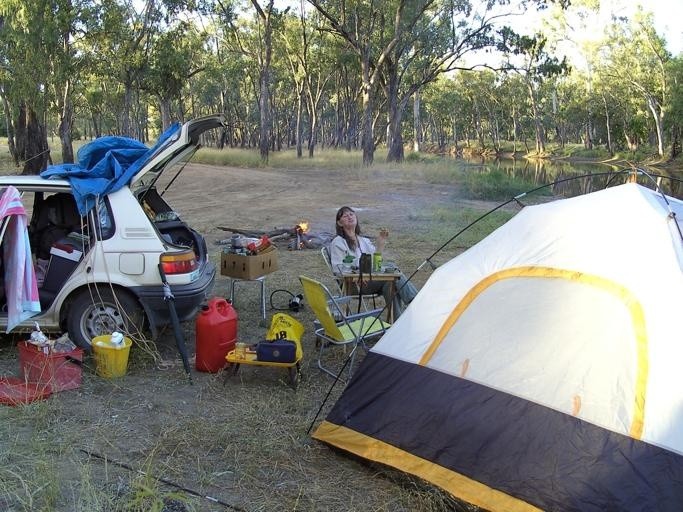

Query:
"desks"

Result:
[[341, 273, 401, 325]]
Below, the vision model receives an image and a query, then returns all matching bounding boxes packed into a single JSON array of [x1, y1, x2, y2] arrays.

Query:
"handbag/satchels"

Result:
[[251, 339, 297, 363]]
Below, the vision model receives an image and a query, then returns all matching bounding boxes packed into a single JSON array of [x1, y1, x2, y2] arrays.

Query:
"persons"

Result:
[[330, 205, 418, 325]]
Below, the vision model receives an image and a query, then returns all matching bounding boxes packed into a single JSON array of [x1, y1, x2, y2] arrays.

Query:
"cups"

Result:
[[230, 235, 240, 246], [234, 342, 245, 361]]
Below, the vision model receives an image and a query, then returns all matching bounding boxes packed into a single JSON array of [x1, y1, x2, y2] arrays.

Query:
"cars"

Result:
[[1, 112, 225, 353]]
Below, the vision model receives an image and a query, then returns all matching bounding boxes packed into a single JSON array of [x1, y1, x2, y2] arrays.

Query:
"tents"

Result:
[[307, 168, 682, 512]]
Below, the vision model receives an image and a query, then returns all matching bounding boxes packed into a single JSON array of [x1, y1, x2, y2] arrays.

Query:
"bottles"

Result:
[[110, 331, 126, 348], [242, 238, 247, 247], [340, 255, 355, 273], [373, 252, 382, 272]]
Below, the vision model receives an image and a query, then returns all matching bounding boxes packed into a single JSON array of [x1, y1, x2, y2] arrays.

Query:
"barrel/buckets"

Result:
[[195, 297, 238, 372], [91, 333, 132, 378]]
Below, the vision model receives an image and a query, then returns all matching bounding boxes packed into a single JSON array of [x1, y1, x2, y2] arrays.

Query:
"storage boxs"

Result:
[[17, 340, 83, 393], [221, 250, 279, 280]]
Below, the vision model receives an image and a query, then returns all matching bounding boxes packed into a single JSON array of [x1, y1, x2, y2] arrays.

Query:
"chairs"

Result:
[[299, 276, 391, 382], [321, 246, 379, 316]]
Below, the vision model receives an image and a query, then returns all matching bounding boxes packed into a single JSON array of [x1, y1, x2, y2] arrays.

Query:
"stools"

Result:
[[231, 276, 266, 319], [222, 349, 302, 393]]
